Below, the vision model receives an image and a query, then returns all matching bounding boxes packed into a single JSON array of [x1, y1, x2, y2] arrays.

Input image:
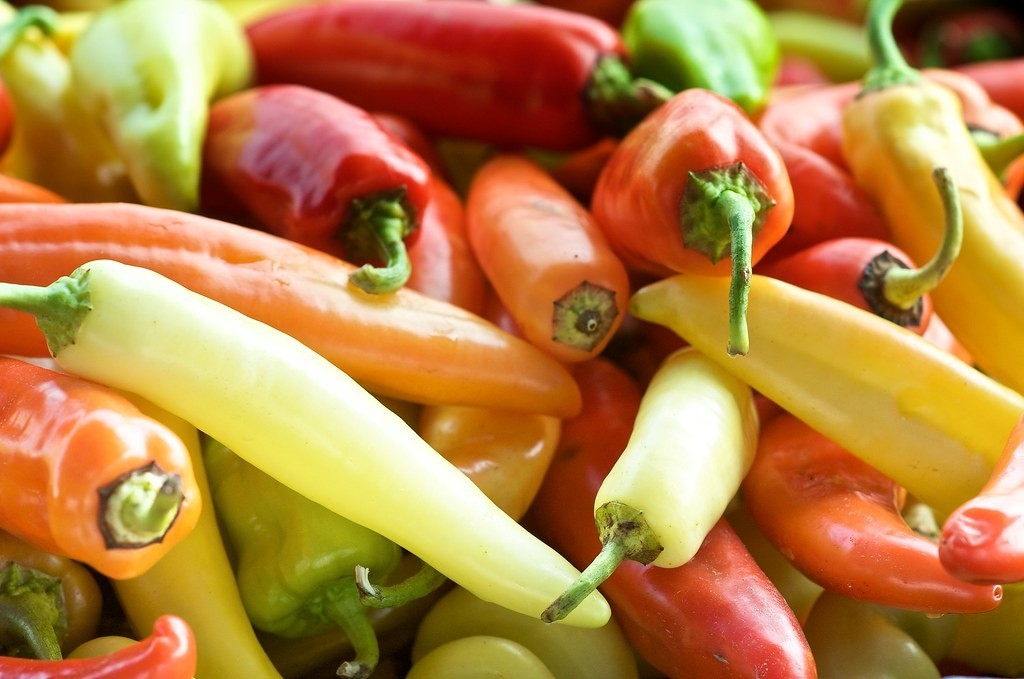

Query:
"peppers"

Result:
[[0, 1, 1024, 678]]
